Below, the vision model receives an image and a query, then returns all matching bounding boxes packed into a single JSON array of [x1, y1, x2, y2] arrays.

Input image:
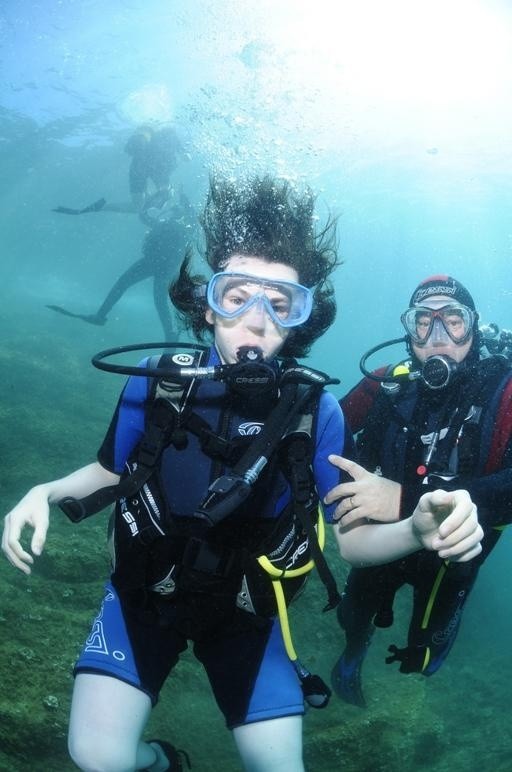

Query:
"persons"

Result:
[[40, 191, 180, 354], [329, 275, 512, 709], [1, 169, 486, 772], [124, 120, 192, 204]]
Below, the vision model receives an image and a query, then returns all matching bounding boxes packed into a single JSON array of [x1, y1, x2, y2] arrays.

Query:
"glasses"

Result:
[[206, 271, 320, 329], [400, 302, 477, 346]]
